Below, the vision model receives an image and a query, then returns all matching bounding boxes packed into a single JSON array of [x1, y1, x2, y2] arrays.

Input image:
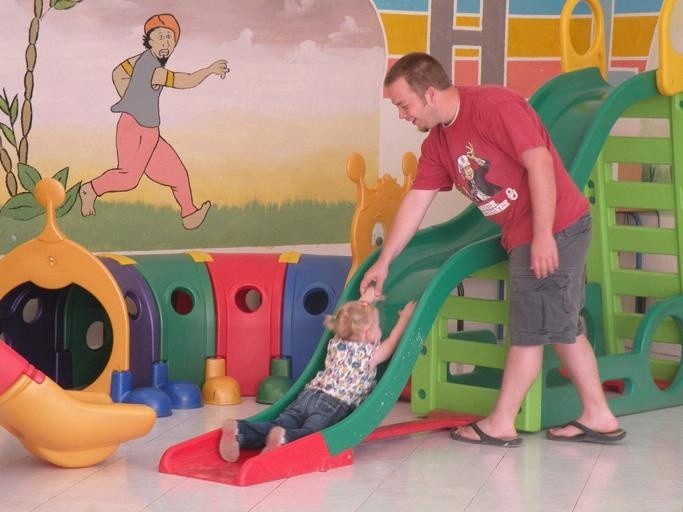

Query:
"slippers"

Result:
[[449, 418, 524, 450], [545, 419, 626, 444]]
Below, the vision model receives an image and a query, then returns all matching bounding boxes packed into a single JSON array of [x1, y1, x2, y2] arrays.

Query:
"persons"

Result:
[[360, 50, 628, 443], [456, 140, 502, 202], [218, 285, 419, 464]]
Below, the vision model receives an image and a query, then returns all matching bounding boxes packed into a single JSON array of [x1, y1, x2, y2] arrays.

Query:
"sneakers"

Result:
[[219, 417, 240, 464], [260, 426, 286, 454]]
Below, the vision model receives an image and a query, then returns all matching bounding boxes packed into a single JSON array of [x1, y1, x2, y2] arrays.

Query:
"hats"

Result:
[[144, 14, 181, 46]]
